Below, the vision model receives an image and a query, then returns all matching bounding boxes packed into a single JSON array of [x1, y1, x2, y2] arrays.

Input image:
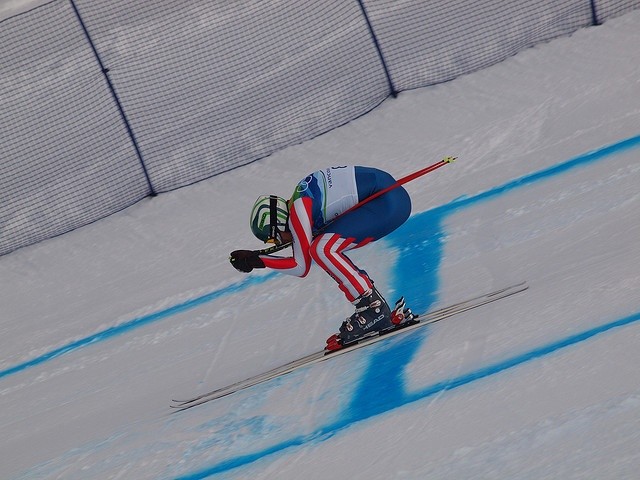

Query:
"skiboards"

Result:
[[170, 281, 529, 410]]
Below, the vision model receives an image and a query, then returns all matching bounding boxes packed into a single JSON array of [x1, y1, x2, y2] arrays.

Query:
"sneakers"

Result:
[[339, 288, 392, 341]]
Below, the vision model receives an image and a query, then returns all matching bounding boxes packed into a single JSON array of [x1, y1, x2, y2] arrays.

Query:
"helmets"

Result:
[[249, 194, 289, 242]]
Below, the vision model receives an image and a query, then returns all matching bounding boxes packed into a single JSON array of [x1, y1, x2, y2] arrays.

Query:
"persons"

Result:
[[229, 164, 412, 340]]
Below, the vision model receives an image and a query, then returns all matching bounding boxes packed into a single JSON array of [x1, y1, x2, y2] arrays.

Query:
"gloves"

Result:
[[229, 249, 266, 274]]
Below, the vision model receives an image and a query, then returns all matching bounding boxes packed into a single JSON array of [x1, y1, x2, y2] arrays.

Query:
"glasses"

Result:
[[263, 235, 274, 244]]
